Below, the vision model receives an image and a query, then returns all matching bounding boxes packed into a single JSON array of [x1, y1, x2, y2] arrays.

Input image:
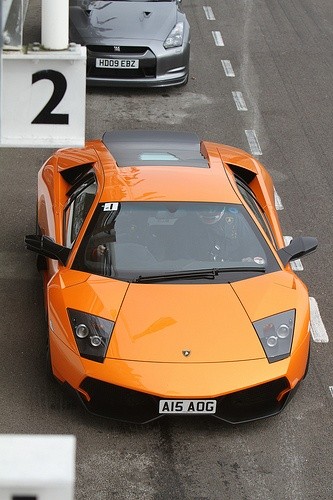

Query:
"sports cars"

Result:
[[23, 128, 318, 426]]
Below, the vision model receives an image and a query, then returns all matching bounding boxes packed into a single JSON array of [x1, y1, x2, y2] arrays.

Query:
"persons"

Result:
[[185, 203, 242, 264]]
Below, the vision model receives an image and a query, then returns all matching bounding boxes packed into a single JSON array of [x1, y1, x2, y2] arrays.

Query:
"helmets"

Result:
[[196, 206, 226, 224]]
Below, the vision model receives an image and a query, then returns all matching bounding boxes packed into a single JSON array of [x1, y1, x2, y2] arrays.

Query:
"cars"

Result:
[[70, 0, 190, 89]]
